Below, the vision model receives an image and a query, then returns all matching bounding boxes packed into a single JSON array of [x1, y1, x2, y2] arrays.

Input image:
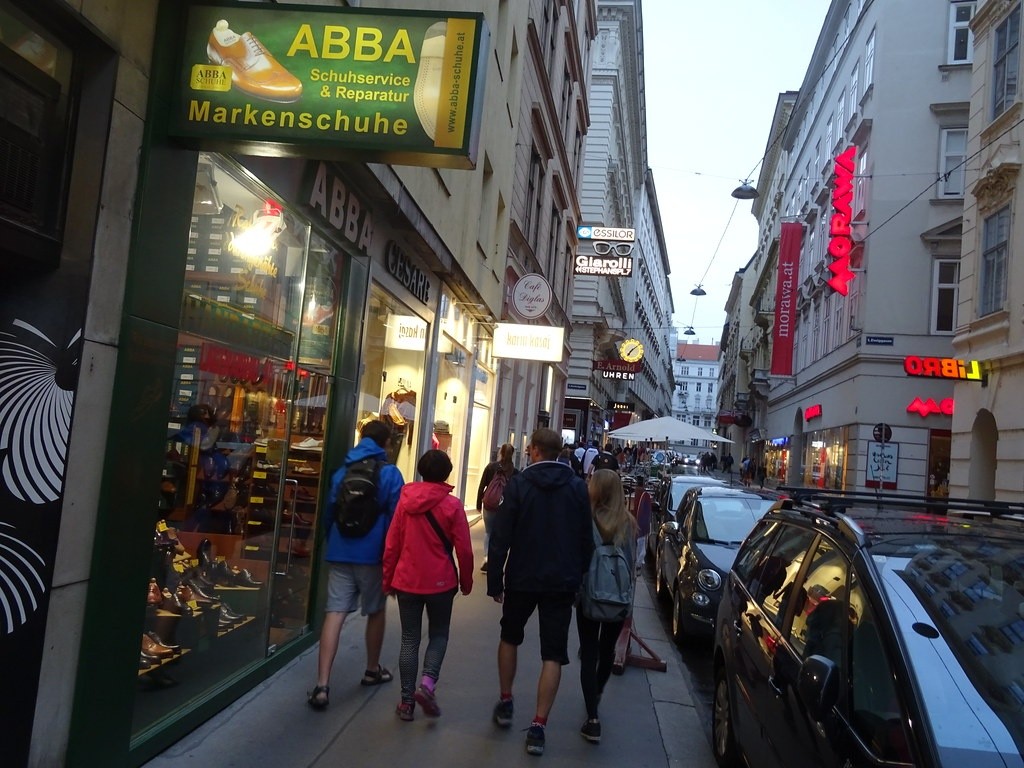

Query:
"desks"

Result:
[[621, 474, 661, 510]]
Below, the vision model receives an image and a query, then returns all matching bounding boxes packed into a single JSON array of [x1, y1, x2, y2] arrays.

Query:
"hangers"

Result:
[[395, 378, 409, 394]]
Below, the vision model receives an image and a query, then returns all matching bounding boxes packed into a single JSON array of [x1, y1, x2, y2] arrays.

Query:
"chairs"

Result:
[[698, 502, 719, 536]]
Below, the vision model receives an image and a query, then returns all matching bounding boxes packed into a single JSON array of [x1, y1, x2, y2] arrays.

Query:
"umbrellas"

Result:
[[610, 416, 735, 474]]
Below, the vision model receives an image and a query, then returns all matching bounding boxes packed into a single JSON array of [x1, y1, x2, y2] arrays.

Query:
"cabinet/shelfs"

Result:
[[162, 271, 327, 630]]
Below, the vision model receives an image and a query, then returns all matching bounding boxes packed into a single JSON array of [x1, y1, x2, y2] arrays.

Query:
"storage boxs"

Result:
[[173, 379, 204, 414], [236, 282, 262, 311], [185, 215, 244, 273], [208, 280, 238, 303], [178, 363, 215, 381], [178, 344, 201, 365], [185, 279, 209, 291]]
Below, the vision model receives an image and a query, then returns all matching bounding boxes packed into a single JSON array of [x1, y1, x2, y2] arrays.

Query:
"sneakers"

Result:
[[494, 698, 515, 723], [396, 700, 416, 721], [520, 725, 545, 754], [253, 435, 283, 447], [581, 720, 601, 741], [257, 460, 280, 472], [292, 462, 320, 475], [291, 436, 324, 451], [415, 684, 440, 716]]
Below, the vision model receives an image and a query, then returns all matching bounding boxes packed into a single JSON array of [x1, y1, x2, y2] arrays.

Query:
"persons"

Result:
[[309, 420, 405, 709], [696, 450, 767, 490], [750, 557, 860, 660], [477, 444, 519, 570], [560, 440, 646, 481], [382, 450, 475, 718], [485, 427, 591, 754], [575, 470, 638, 741]]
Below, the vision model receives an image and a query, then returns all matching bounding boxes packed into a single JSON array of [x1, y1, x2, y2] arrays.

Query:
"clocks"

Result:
[[618, 339, 644, 363]]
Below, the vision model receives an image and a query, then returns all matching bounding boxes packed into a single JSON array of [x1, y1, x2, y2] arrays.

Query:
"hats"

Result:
[[187, 404, 218, 428], [167, 421, 208, 446]]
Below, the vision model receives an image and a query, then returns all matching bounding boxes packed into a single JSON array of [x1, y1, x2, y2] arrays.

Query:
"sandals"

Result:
[[308, 684, 329, 708], [361, 663, 393, 684]]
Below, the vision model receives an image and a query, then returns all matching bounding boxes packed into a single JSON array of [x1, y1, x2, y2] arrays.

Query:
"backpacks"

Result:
[[580, 519, 633, 621], [483, 467, 509, 512], [332, 462, 388, 539]]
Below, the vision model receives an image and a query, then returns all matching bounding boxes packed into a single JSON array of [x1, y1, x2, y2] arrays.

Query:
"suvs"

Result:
[[710, 485, 1020, 765]]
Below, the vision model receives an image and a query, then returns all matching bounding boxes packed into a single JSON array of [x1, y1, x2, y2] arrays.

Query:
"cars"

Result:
[[655, 487, 773, 643], [801, 462, 842, 488], [645, 448, 731, 574]]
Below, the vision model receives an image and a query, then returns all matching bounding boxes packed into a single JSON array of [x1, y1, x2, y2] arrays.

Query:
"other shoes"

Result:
[[481, 562, 488, 573]]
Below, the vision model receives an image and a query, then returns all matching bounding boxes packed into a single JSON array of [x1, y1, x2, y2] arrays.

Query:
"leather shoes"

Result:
[[137, 361, 317, 668]]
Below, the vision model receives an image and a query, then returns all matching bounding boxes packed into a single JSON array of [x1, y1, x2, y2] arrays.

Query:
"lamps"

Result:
[[676, 357, 686, 361], [233, 204, 287, 256], [690, 283, 706, 295], [192, 157, 225, 215], [732, 179, 760, 199], [684, 327, 695, 335]]
